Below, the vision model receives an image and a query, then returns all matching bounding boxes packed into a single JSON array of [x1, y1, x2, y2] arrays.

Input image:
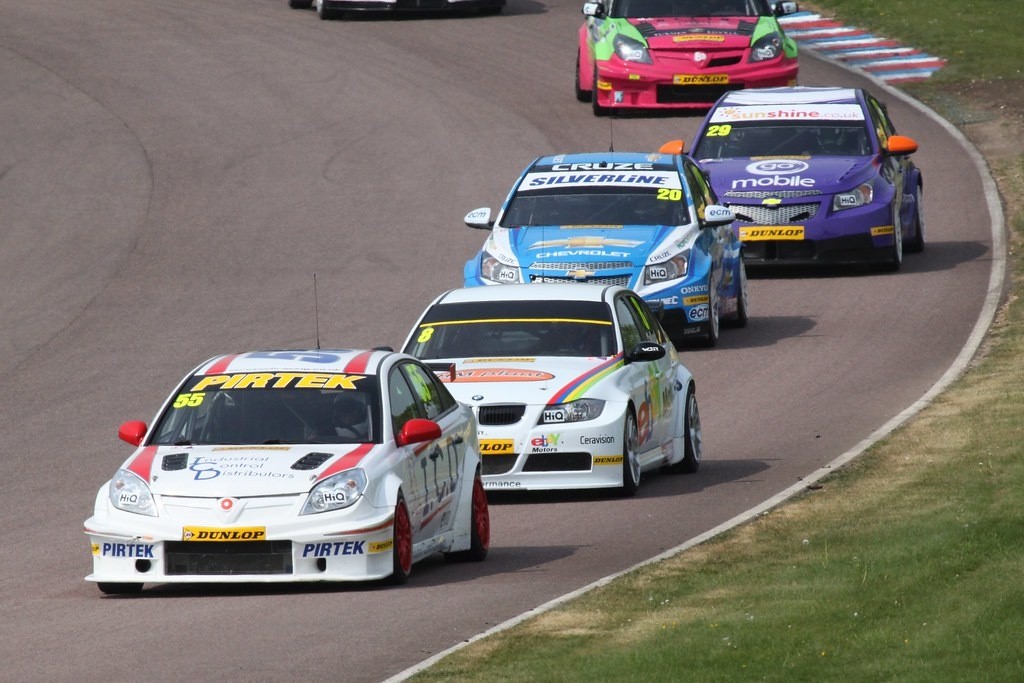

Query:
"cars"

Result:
[[656, 83, 928, 274], [575, 0, 802, 116], [84, 348, 489, 593], [395, 284, 703, 501], [460, 141, 747, 347]]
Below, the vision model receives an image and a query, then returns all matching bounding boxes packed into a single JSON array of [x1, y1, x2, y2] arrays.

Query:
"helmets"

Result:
[[629, 194, 668, 222], [551, 302, 597, 355], [332, 392, 368, 439], [815, 128, 847, 150]]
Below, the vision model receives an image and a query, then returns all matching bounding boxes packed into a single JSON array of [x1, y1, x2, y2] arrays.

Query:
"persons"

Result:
[[330, 391, 370, 440]]
